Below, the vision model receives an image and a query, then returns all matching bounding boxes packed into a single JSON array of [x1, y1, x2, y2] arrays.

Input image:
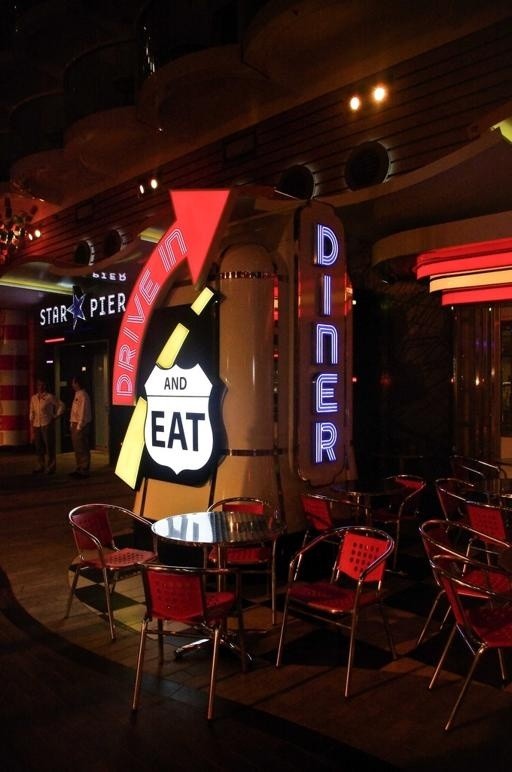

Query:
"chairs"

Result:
[[273, 526, 395, 697], [295, 491, 364, 589], [203, 497, 282, 632], [434, 475, 511, 586], [128, 559, 240, 726], [368, 471, 427, 582], [418, 518, 510, 662], [427, 554, 511, 735], [457, 455, 510, 541], [66, 502, 158, 642]]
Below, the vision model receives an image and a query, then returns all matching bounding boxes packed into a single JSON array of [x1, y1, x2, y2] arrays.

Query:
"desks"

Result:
[[331, 478, 401, 575], [151, 512, 285, 668]]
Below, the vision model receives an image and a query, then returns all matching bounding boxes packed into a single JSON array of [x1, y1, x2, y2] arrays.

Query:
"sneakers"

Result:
[[31, 465, 90, 478]]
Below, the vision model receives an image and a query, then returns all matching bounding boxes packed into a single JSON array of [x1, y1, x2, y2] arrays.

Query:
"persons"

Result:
[[26, 376, 66, 477], [67, 373, 93, 480]]
